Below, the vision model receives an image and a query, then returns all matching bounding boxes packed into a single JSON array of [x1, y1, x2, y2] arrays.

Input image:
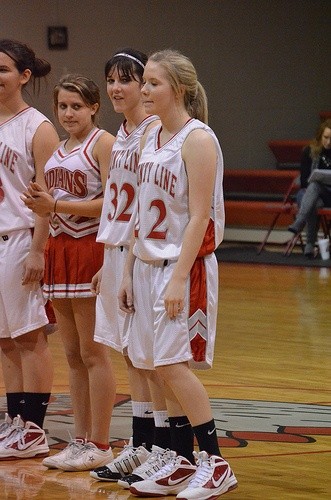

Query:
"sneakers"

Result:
[[0, 413, 50, 461], [129, 455, 198, 497], [54, 442, 114, 472], [89, 441, 152, 481], [118, 445, 177, 488], [176, 450, 238, 500], [42, 431, 88, 468]]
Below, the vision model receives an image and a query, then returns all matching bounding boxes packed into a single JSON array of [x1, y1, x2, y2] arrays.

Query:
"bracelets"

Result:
[[54, 200, 57, 214]]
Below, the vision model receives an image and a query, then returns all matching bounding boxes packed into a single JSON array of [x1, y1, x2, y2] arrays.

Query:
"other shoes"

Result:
[[287, 215, 306, 233], [304, 243, 315, 260]]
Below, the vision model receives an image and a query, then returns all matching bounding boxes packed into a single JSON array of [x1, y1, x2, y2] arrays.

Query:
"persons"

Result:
[[89, 48, 238, 500], [0, 39, 60, 460], [287, 119, 331, 257], [20, 75, 117, 472]]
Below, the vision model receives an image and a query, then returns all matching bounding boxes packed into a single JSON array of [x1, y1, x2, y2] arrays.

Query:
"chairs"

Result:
[[256, 169, 331, 258]]
